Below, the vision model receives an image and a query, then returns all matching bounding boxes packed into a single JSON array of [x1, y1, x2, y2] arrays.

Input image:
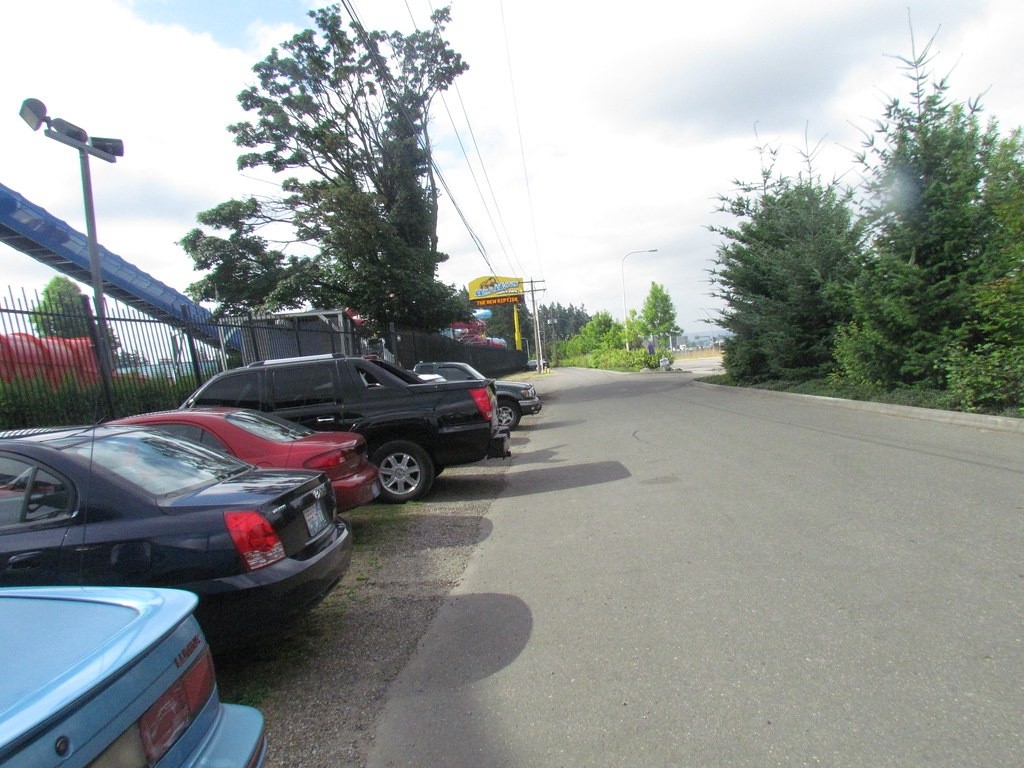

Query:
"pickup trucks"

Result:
[[400, 361, 540, 429], [174, 353, 511, 508]]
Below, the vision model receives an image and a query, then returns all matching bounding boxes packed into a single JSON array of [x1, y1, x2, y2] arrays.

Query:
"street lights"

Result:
[[521, 338, 530, 362], [622, 249, 657, 349], [19, 97, 125, 422]]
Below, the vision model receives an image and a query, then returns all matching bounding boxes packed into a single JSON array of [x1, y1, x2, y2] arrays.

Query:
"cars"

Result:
[[521, 359, 546, 373], [0, 426, 353, 655], [0, 407, 382, 514], [1, 584, 266, 768]]
